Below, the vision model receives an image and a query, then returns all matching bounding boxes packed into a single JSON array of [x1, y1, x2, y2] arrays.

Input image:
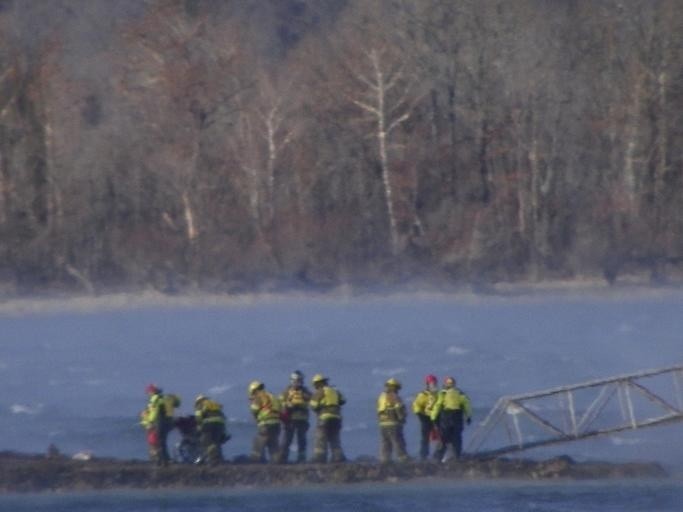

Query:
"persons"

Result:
[[308, 373, 346, 463], [277, 370, 312, 463], [431, 376, 473, 460], [246, 381, 282, 463], [140, 384, 181, 465], [375, 376, 409, 463], [410, 374, 440, 460], [193, 394, 227, 466]]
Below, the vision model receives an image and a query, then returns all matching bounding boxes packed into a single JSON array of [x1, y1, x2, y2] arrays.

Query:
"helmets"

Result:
[[246, 371, 329, 397], [383, 375, 456, 388], [145, 384, 208, 406]]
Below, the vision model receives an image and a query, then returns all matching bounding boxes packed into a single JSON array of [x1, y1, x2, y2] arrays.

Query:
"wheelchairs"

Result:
[[173, 416, 225, 464]]
